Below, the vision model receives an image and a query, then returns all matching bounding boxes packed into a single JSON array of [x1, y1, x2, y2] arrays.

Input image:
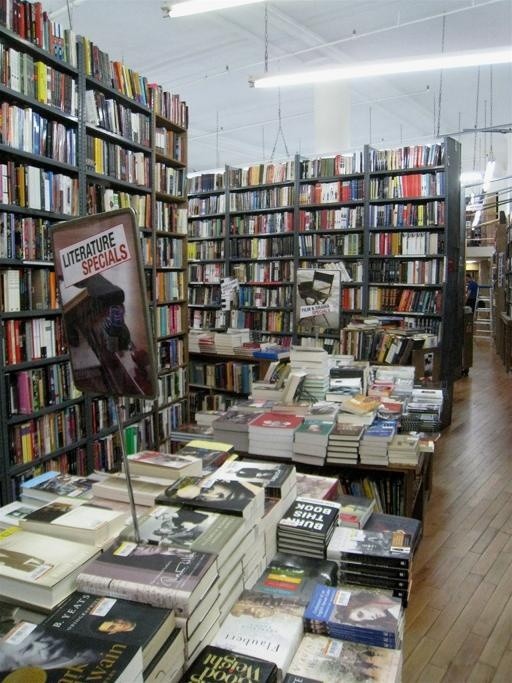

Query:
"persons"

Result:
[[465, 273, 478, 316]]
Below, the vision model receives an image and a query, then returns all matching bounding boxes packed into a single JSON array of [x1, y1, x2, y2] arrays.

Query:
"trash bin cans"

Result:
[[461, 306, 474, 377]]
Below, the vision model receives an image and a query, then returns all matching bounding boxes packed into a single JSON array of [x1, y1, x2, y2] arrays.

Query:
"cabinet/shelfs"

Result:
[[183, 132, 461, 378], [0, 16, 184, 508]]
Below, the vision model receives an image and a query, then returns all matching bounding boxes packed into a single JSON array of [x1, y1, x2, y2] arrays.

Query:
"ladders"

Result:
[[472, 285, 496, 338]]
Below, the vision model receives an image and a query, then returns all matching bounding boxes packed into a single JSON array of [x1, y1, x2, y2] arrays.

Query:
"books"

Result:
[[0, 0, 188, 370], [187, 143, 446, 332], [2, 326, 445, 683], [491, 229, 512, 333]]
